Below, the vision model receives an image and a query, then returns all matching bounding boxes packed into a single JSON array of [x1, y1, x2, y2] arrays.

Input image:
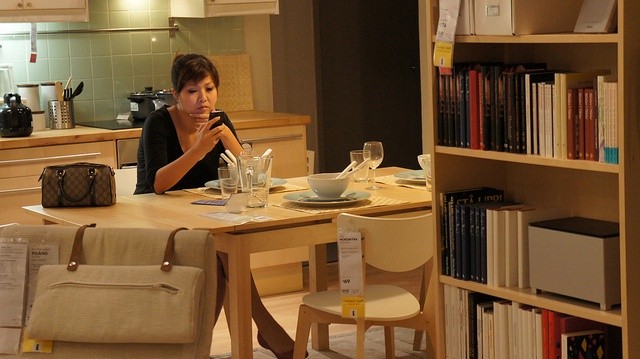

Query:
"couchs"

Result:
[[1, 221, 213, 358]]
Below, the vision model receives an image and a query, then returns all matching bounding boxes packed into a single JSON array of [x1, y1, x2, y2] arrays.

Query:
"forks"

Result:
[[64, 88, 72, 101]]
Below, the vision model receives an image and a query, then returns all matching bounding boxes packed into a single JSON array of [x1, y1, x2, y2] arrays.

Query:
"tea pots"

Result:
[[0, 92, 33, 137]]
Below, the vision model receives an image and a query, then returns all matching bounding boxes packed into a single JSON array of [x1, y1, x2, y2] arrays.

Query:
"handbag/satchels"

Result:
[[38, 162, 117, 207], [25, 223, 205, 342]]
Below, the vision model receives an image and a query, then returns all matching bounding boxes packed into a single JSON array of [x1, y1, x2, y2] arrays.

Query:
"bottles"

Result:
[[17, 83, 40, 113], [40, 82, 62, 112]]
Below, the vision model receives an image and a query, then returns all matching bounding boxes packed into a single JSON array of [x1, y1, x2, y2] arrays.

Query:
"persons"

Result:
[[132, 53, 309, 359]]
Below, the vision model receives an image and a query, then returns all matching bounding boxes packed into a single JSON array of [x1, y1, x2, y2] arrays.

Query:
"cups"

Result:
[[218, 166, 239, 199], [237, 157, 272, 207], [350, 150, 371, 181]]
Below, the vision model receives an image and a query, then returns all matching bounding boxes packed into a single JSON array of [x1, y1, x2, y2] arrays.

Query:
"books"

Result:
[[439, 188, 528, 289], [444, 285, 611, 359], [430, 61, 618, 164]]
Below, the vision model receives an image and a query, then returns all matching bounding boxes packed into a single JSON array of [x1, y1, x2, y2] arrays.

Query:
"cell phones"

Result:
[[209, 112, 223, 131]]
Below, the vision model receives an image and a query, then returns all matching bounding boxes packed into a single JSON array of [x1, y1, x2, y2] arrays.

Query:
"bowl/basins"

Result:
[[307, 173, 350, 199], [418, 154, 432, 179]]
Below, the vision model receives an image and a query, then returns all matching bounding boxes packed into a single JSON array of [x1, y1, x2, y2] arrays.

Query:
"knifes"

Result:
[[70, 81, 84, 101]]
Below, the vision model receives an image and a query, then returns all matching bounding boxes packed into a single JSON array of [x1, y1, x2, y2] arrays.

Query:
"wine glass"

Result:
[[363, 141, 384, 191]]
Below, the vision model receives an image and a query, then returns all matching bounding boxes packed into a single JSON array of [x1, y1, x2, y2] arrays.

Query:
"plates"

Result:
[[204, 177, 288, 191], [283, 190, 371, 206], [394, 170, 426, 185]]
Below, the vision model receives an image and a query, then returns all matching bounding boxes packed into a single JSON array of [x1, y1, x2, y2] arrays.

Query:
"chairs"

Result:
[[113, 168, 139, 195], [294, 213, 432, 359]]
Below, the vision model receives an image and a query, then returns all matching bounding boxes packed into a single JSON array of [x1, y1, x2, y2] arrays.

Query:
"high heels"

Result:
[[257, 330, 309, 359]]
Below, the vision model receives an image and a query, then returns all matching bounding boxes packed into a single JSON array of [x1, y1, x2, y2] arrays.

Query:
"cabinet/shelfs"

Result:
[[232, 126, 309, 270], [418, 0, 640, 358], [0, 139, 118, 229]]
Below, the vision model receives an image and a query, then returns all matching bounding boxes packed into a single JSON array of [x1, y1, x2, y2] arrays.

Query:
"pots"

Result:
[[152, 89, 176, 110], [127, 86, 157, 123]]
